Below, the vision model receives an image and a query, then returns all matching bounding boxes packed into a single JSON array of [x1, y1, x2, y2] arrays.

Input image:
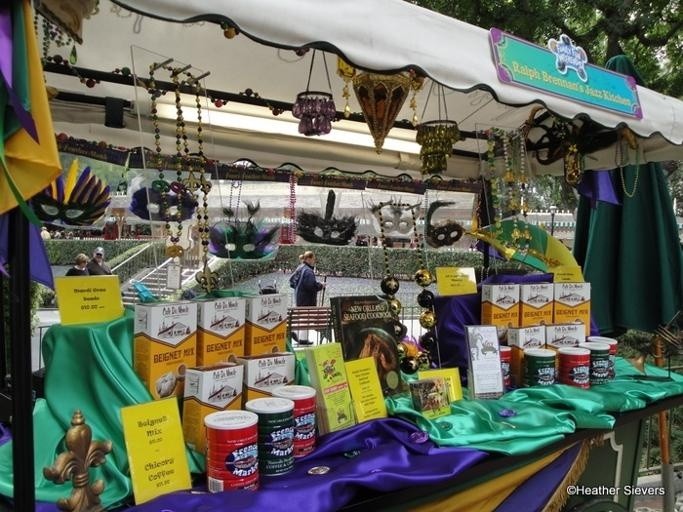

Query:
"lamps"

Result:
[[414, 80, 460, 175], [292, 47, 339, 135], [334, 58, 425, 156]]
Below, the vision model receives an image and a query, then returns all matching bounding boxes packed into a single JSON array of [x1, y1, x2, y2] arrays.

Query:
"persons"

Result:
[[85, 246, 113, 276], [296, 254, 305, 267], [289, 250, 326, 347], [63, 253, 89, 277]]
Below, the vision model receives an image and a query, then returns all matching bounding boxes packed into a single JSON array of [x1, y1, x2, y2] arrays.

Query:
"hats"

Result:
[[94, 247, 104, 255]]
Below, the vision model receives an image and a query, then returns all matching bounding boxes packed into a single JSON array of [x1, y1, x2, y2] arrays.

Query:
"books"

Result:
[[304, 342, 357, 436]]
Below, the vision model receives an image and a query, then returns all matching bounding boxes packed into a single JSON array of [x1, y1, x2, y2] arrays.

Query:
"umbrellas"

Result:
[[582, 41, 683, 510], [460, 215, 589, 289]]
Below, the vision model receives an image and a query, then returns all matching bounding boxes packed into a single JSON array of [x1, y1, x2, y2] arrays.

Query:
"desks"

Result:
[[338, 392, 682, 512]]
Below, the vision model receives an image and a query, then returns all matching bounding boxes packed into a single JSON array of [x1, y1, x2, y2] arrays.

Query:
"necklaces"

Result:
[[171, 66, 222, 295], [618, 139, 642, 199], [145, 63, 196, 266], [478, 124, 535, 258], [375, 198, 440, 377], [286, 173, 299, 244]]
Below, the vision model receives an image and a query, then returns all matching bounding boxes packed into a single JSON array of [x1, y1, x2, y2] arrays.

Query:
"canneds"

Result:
[[499, 345, 512, 389], [204, 385, 318, 493], [523, 335, 618, 390]]
